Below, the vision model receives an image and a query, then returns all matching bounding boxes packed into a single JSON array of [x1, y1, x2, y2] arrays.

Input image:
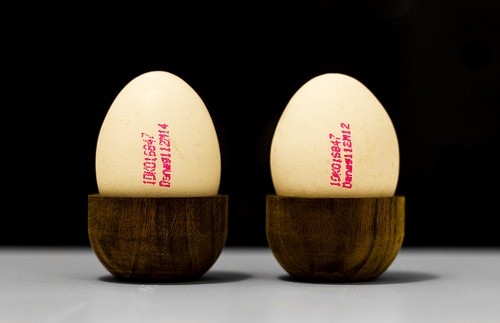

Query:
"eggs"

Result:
[[94, 70, 222, 197], [270, 73, 401, 197]]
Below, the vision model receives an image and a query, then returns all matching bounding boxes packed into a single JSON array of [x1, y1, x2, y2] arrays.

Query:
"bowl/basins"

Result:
[[88, 194, 229, 283], [265, 195, 406, 286]]
